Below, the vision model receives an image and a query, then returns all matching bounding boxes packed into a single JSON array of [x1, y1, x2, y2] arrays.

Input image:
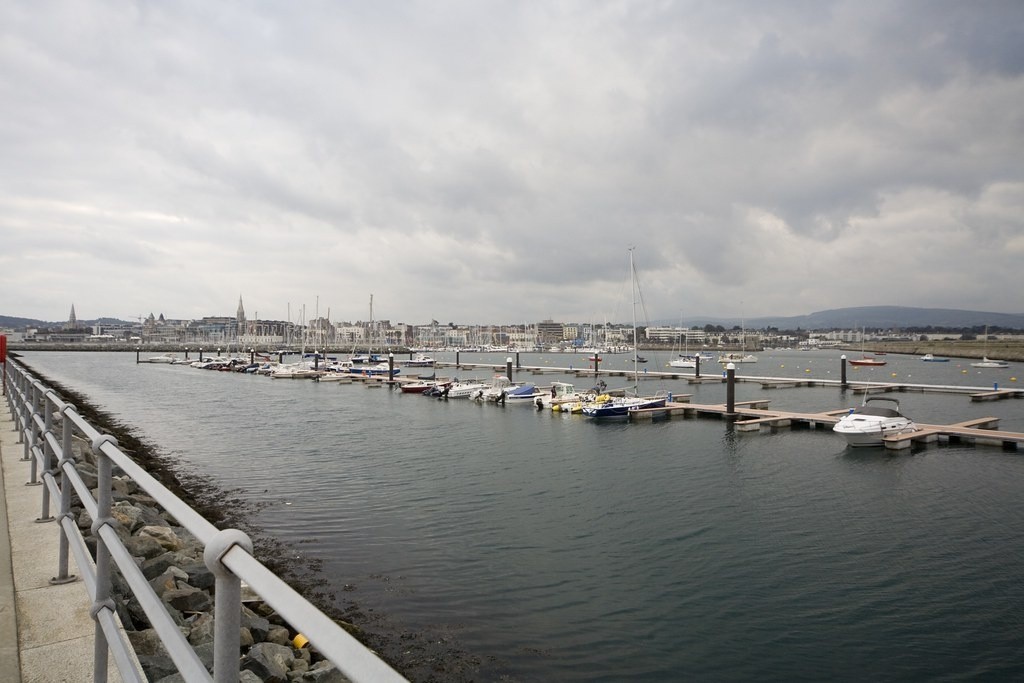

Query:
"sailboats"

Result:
[[666, 311, 696, 368], [716, 315, 758, 363], [575, 244, 665, 427]]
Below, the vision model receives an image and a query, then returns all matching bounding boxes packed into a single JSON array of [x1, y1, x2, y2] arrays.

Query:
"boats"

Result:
[[631, 356, 648, 363], [848, 356, 889, 367], [587, 357, 602, 362], [681, 351, 714, 362], [151, 356, 624, 413], [833, 397, 919, 447], [921, 352, 953, 362], [971, 361, 1013, 368]]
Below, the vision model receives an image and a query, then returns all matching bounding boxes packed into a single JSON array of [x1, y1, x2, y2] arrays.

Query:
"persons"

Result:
[[551, 386, 557, 399]]
